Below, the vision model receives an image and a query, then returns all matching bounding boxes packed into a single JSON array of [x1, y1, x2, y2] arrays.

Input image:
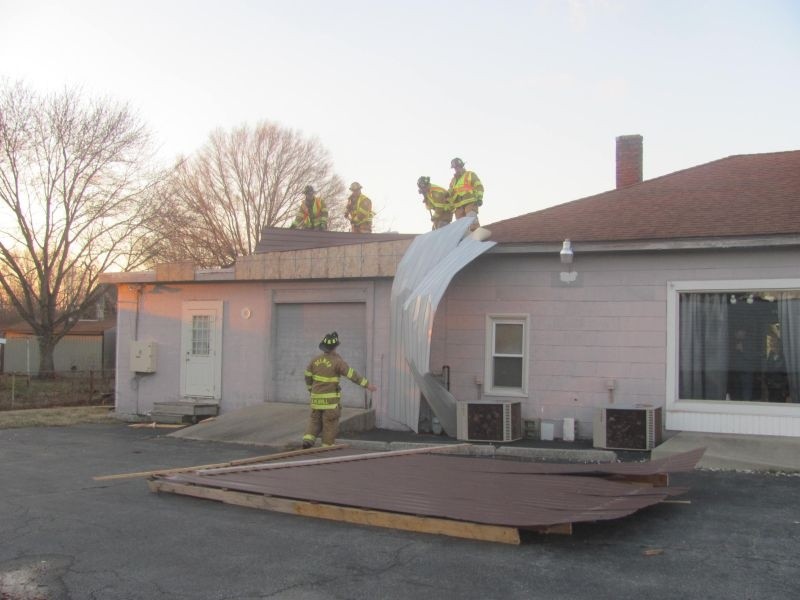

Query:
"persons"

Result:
[[418, 176, 452, 230], [734, 329, 753, 399], [291, 186, 328, 231], [303, 331, 376, 450], [344, 182, 372, 233], [766, 338, 790, 402], [445, 158, 485, 232]]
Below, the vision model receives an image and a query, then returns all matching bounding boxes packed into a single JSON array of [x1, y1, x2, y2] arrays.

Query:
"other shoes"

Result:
[[302, 442, 311, 449]]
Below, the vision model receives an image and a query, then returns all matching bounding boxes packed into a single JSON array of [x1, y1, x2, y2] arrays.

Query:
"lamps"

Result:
[[747, 295, 754, 304], [730, 295, 736, 303], [560, 240, 572, 263]]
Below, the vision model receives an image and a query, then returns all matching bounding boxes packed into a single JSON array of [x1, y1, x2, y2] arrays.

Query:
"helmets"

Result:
[[451, 158, 464, 168], [417, 176, 430, 189], [349, 182, 363, 191], [319, 332, 340, 351], [302, 185, 313, 193]]
